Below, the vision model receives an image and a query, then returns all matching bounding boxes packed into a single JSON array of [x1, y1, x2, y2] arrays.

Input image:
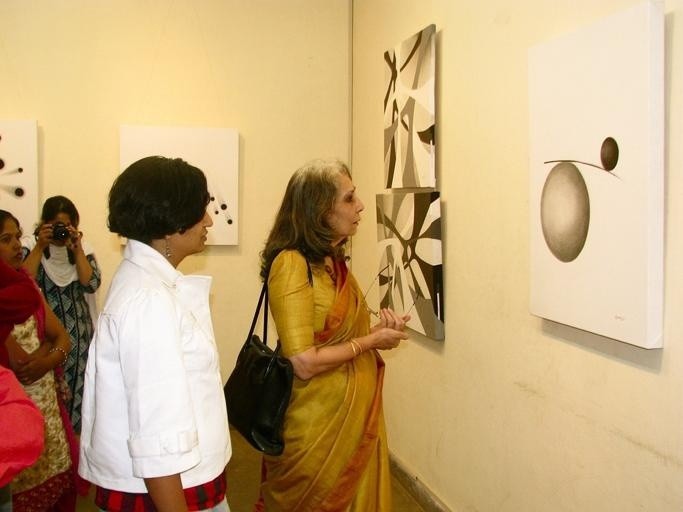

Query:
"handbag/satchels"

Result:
[[223, 244, 313, 456]]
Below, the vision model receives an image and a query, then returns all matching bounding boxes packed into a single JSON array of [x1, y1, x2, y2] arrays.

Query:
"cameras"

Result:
[[51, 221, 69, 244]]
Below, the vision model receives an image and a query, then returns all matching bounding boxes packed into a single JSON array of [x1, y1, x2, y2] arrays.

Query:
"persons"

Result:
[[21, 194, 102, 434], [78, 155, 235, 512], [254, 158, 412, 512], [1, 211, 85, 512]]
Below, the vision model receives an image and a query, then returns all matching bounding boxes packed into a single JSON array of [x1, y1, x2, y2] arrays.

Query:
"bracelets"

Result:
[[353, 338, 362, 353], [350, 342, 358, 359], [49, 347, 68, 368]]
